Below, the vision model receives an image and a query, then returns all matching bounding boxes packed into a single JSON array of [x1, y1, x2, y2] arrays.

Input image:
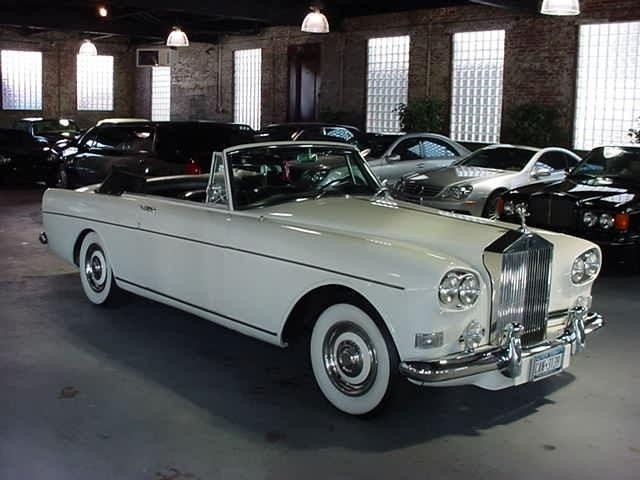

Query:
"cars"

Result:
[[39, 140, 603, 419]]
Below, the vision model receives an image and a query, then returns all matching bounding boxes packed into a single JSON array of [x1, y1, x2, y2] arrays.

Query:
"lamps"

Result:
[[301, 4, 330, 34], [78, 39, 97, 55], [166, 23, 189, 48], [538, 0, 582, 17]]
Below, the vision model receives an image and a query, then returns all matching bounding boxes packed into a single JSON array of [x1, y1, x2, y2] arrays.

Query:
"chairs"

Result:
[[183, 185, 272, 207]]
